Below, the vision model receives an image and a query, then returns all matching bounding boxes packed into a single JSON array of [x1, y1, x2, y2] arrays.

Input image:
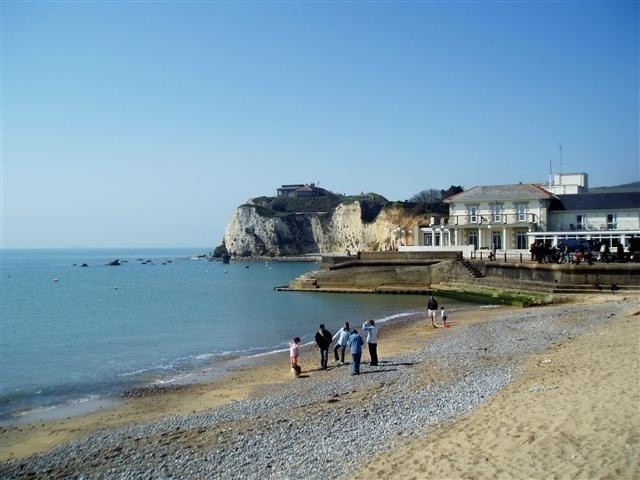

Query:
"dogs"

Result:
[[289, 363, 301, 380]]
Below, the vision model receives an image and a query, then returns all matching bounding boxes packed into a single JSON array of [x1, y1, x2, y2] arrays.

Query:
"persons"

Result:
[[288, 336, 301, 365], [488, 242, 496, 260], [362, 319, 379, 366], [427, 296, 438, 325], [440, 306, 447, 329], [346, 330, 364, 375], [315, 324, 332, 370], [529, 239, 634, 265], [332, 321, 352, 365]]
